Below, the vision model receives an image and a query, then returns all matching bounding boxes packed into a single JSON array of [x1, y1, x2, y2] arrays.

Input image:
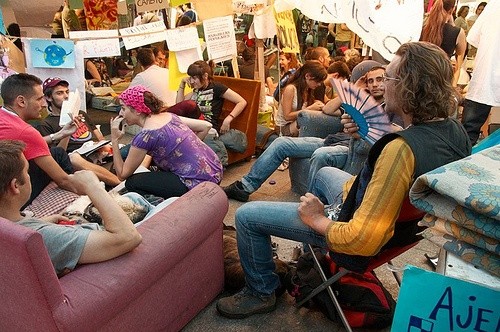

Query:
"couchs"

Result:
[[0, 181, 226, 332]]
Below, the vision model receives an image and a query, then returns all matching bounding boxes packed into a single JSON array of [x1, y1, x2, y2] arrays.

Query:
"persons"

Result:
[[216, 41, 472, 318], [0, 137, 144, 280], [1, 4, 403, 200], [418, 0, 500, 146]]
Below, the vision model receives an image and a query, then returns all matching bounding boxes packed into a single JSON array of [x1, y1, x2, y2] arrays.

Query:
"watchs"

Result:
[[50, 134, 56, 143]]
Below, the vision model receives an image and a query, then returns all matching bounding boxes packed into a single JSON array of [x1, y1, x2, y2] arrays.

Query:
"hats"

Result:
[[43, 76, 69, 92]]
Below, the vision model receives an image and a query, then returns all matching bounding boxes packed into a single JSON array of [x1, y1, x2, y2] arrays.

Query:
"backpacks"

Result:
[[290, 249, 397, 332]]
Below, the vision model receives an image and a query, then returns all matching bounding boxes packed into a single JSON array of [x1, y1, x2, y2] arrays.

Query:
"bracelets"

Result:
[[229, 114, 235, 119]]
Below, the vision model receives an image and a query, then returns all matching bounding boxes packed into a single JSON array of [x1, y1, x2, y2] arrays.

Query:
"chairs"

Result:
[[294, 193, 426, 332], [212, 74, 262, 164]]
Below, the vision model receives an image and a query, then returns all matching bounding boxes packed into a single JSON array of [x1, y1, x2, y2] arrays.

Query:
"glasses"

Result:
[[381, 73, 401, 82]]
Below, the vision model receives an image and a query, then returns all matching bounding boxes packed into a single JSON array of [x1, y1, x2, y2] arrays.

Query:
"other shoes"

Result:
[[216, 287, 277, 319], [219, 181, 249, 203]]
